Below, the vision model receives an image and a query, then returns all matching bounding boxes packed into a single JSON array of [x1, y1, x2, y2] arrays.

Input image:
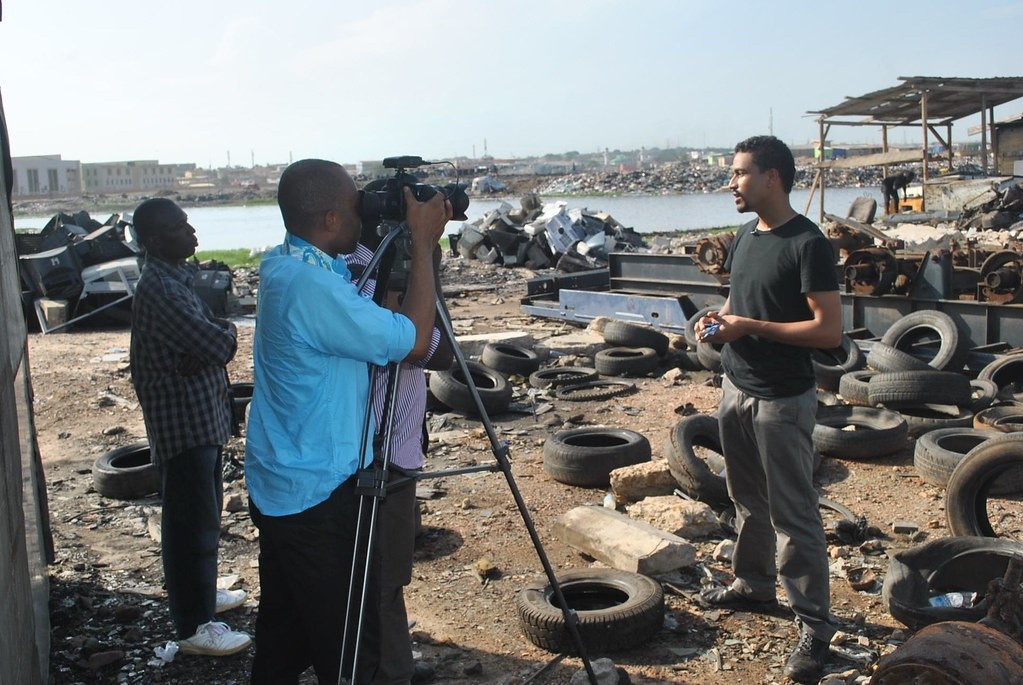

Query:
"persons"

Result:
[[345, 179, 456, 685], [243, 159, 457, 684], [132, 198, 252, 659], [693, 137, 844, 681], [881, 172, 914, 216]]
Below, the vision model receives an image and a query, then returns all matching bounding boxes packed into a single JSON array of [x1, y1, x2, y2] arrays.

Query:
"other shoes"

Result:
[[412, 661, 436, 683]]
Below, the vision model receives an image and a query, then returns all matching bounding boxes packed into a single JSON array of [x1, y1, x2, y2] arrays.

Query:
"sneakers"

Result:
[[213, 588, 247, 615], [180, 620, 251, 656]]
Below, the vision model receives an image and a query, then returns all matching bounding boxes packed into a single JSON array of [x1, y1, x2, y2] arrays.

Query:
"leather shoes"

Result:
[[700, 585, 778, 613], [785, 630, 829, 681]]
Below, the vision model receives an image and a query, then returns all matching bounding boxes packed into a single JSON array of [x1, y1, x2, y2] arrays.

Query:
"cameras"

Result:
[[355, 156, 470, 222]]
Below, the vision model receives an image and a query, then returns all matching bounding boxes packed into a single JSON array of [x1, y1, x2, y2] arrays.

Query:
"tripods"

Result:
[[338, 224, 598, 685]]
